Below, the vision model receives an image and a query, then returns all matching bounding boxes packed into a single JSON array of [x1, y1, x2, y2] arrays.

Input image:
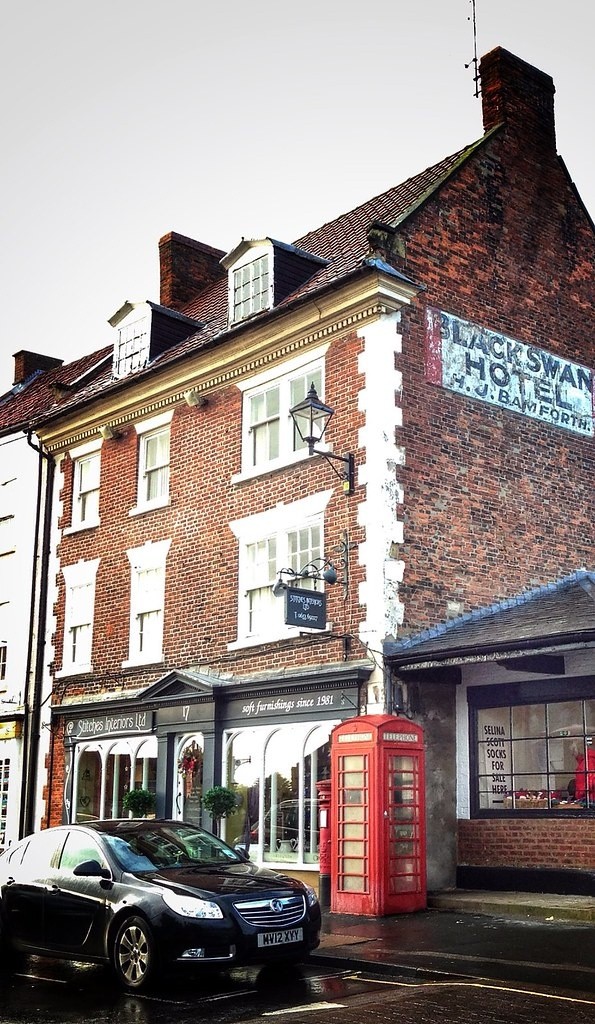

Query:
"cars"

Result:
[[233, 798, 323, 846], [0, 817, 321, 993]]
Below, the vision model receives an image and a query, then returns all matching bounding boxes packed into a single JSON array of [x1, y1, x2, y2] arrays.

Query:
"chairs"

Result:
[[136, 836, 170, 867]]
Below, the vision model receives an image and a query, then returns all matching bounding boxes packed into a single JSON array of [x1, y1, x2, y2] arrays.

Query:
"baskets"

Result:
[[503, 798, 547, 809]]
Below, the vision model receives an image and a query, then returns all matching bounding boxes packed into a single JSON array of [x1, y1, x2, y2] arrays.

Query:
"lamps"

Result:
[[288, 382, 355, 496], [99, 425, 123, 441], [272, 530, 348, 601], [184, 389, 208, 409]]
